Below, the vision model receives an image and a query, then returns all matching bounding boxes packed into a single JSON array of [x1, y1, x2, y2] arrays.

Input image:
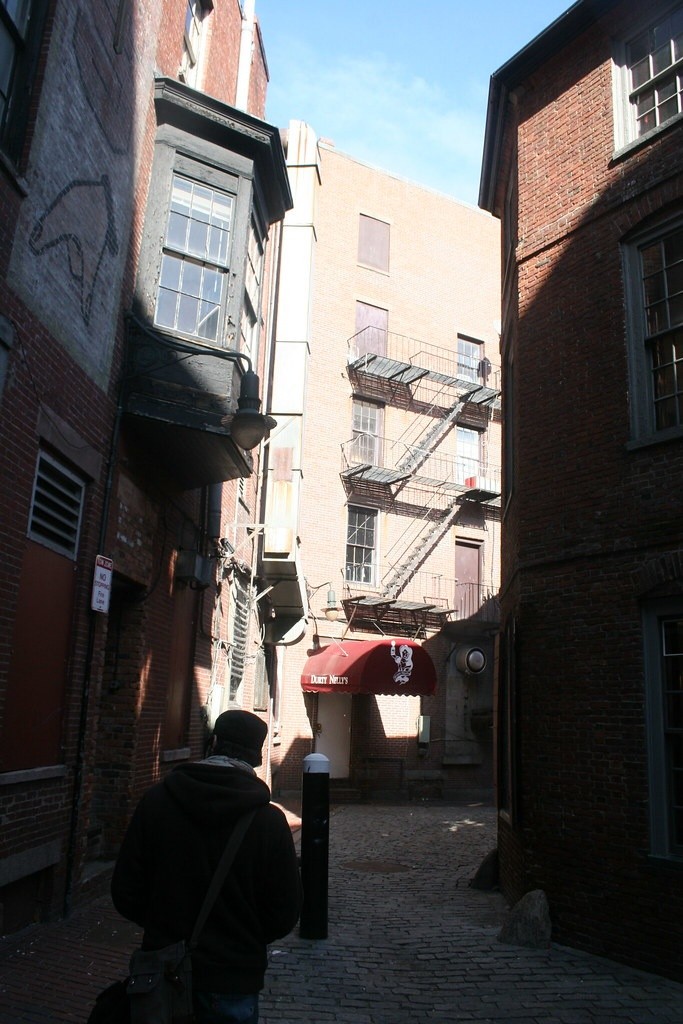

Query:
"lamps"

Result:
[[305, 579, 342, 623]]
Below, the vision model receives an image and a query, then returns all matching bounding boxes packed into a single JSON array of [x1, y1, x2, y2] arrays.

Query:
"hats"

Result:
[[212, 710, 267, 750]]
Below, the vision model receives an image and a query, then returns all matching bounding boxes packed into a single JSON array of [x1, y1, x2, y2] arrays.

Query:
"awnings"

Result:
[[300, 638, 437, 696]]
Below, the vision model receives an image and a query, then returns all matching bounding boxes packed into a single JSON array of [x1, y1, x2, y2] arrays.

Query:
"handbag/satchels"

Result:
[[126, 940, 193, 1024]]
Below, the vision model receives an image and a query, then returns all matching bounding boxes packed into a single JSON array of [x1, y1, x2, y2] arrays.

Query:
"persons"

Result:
[[110, 709, 303, 1024]]
